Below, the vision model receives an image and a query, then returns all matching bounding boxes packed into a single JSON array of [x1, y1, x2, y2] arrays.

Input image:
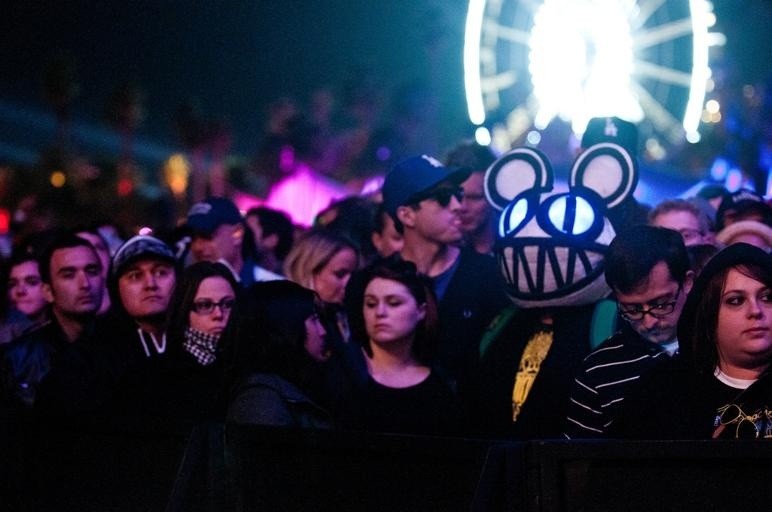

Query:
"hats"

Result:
[[381, 152, 473, 203], [176, 196, 244, 240], [111, 235, 175, 280], [715, 187, 769, 215], [714, 219, 772, 249]]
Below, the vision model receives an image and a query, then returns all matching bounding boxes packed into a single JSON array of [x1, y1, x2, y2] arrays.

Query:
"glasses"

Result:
[[405, 187, 466, 208], [187, 295, 234, 316], [615, 277, 685, 322]]
